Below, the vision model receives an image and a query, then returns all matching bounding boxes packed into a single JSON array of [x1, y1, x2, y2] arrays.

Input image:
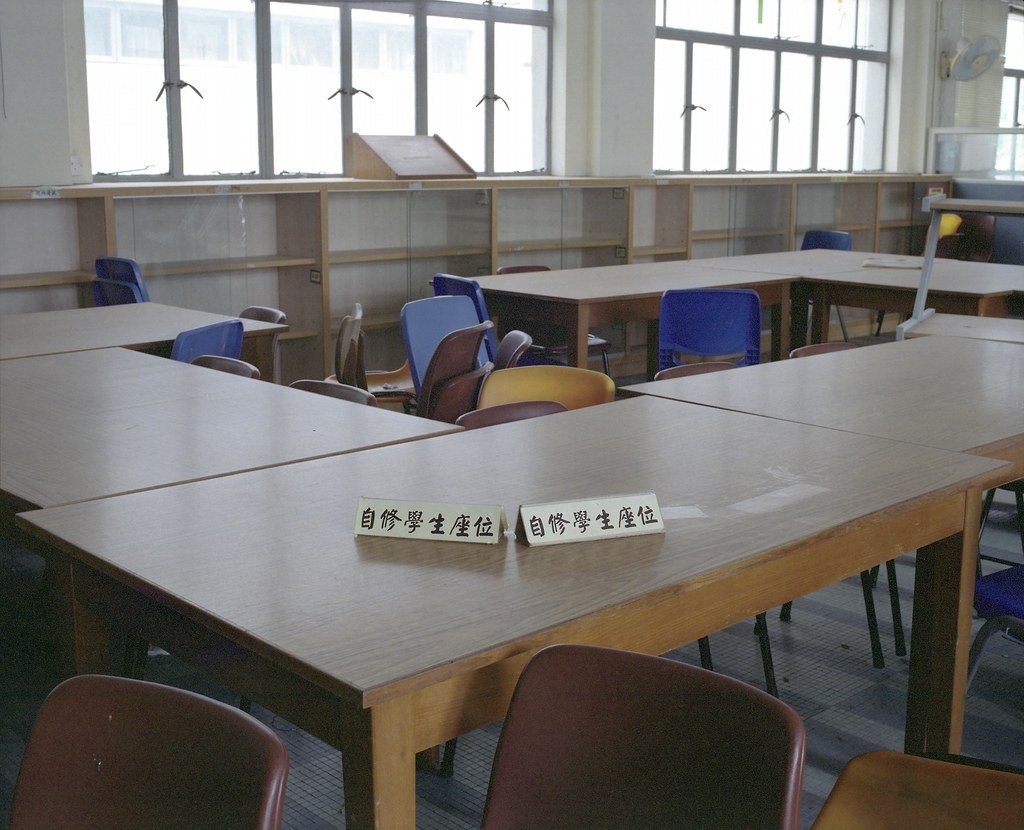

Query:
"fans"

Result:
[[939, 32, 1001, 83]]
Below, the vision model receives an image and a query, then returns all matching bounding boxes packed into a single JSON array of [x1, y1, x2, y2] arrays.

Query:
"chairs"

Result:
[[85, 220, 1024, 759], [7, 676, 288, 830], [812, 750, 1024, 829], [961, 563, 1023, 697], [482, 643, 807, 829]]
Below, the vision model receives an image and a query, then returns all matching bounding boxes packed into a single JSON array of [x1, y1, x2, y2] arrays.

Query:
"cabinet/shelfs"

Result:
[[1, 172, 957, 385]]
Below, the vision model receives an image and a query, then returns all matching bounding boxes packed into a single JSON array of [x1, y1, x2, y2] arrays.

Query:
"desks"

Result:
[[0, 249, 1024, 830]]
